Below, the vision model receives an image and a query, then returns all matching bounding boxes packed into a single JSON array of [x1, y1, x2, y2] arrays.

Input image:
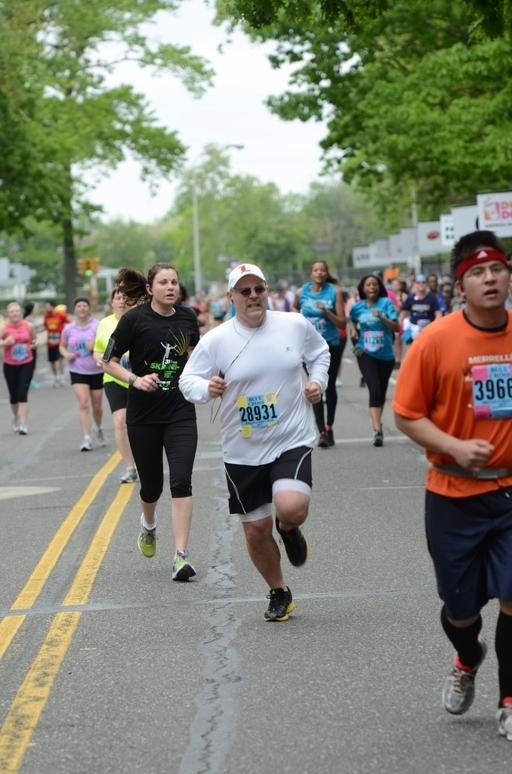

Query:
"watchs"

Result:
[[129, 374, 139, 386]]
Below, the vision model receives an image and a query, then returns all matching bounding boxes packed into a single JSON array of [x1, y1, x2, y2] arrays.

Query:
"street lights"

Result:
[[191, 143, 244, 295]]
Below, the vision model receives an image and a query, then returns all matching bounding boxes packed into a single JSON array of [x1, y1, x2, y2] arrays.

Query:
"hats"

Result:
[[228, 263, 265, 291]]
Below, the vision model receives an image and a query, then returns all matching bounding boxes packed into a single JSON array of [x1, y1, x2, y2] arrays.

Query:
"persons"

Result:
[[292, 261, 348, 448], [392, 231, 512, 743], [177, 263, 331, 621], [179, 285, 296, 336], [349, 274, 400, 447], [23, 303, 41, 389], [335, 250, 512, 391], [59, 296, 104, 453], [92, 287, 140, 485], [42, 300, 71, 386], [99, 261, 201, 582], [0, 300, 39, 435]]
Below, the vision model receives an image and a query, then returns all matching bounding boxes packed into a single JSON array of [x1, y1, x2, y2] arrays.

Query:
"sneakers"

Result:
[[264, 586, 294, 622], [91, 423, 107, 447], [318, 430, 334, 447], [443, 637, 486, 712], [171, 549, 197, 580], [11, 423, 28, 435], [79, 438, 92, 452], [120, 468, 136, 483], [372, 422, 385, 445], [273, 515, 308, 567], [492, 698, 510, 744], [137, 513, 158, 557]]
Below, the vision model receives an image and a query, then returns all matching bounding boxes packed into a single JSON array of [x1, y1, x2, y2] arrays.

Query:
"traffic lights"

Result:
[[84, 257, 94, 276], [77, 258, 85, 276], [92, 256, 100, 274]]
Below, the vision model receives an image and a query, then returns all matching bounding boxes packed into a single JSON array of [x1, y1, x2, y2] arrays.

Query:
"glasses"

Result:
[[240, 286, 266, 296]]
